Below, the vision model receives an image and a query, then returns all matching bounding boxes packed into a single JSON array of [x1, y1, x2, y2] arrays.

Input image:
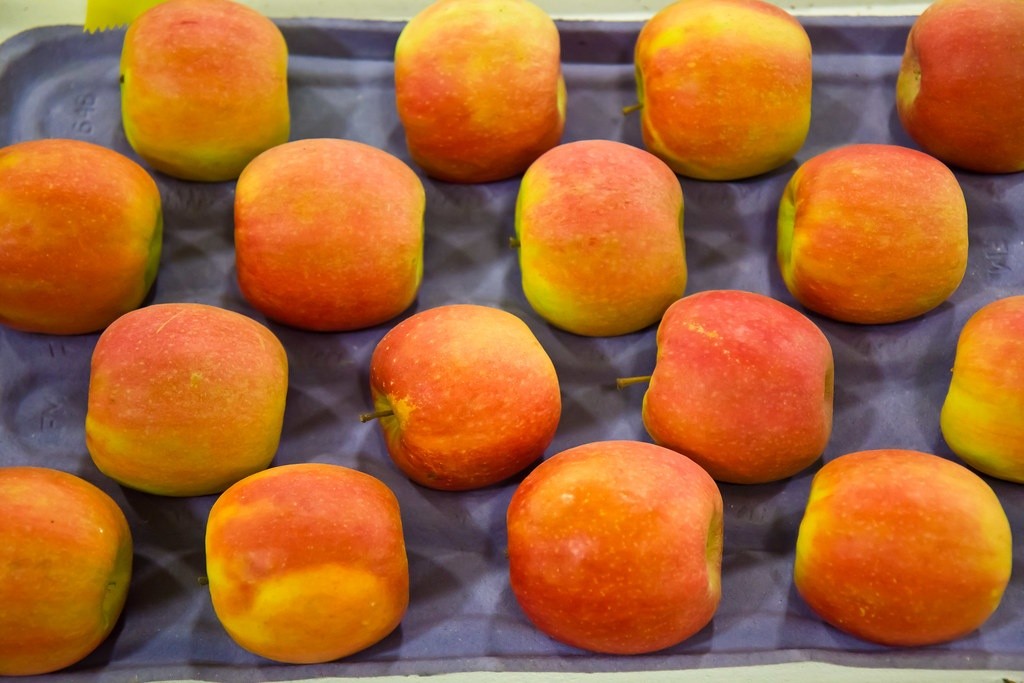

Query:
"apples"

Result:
[[116, 0, 290, 183], [197, 462, 410, 666], [84, 304, 289, 497], [896, 0, 1024, 174], [775, 142, 970, 321], [792, 450, 1014, 646], [235, 138, 426, 331], [502, 440, 724, 654], [939, 295, 1024, 484], [0, 464, 134, 676], [358, 305, 561, 492], [393, 0, 569, 185], [615, 288, 836, 483], [0, 138, 164, 334], [509, 139, 687, 336], [623, 0, 812, 179]]
